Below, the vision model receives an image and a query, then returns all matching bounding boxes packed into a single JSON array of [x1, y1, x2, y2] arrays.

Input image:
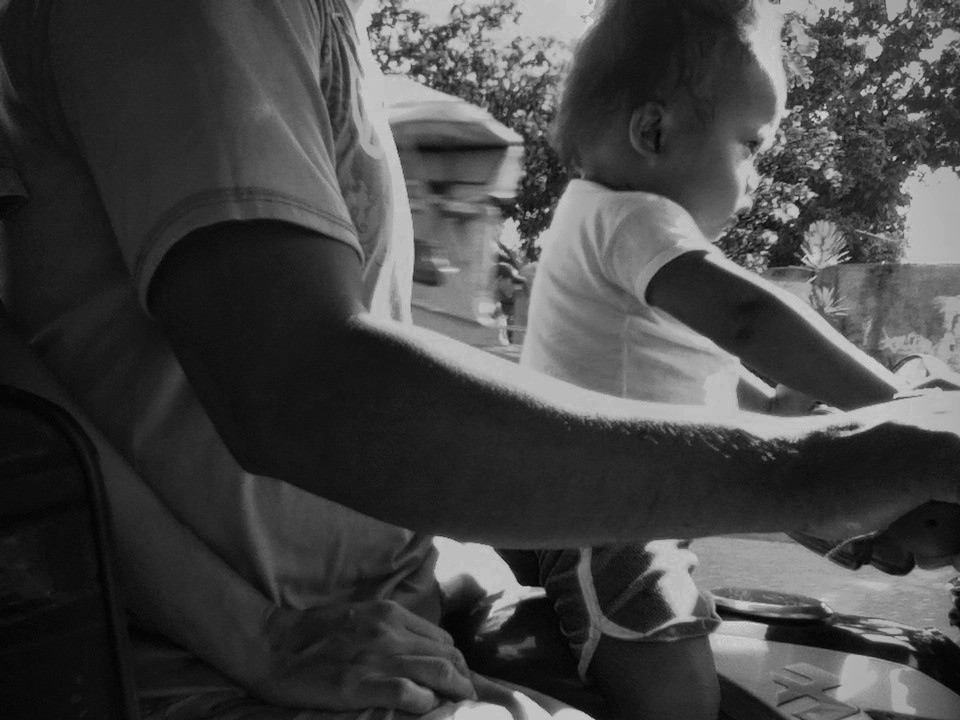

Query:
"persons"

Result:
[[517, 1, 910, 718], [1, 1, 960, 719]]
